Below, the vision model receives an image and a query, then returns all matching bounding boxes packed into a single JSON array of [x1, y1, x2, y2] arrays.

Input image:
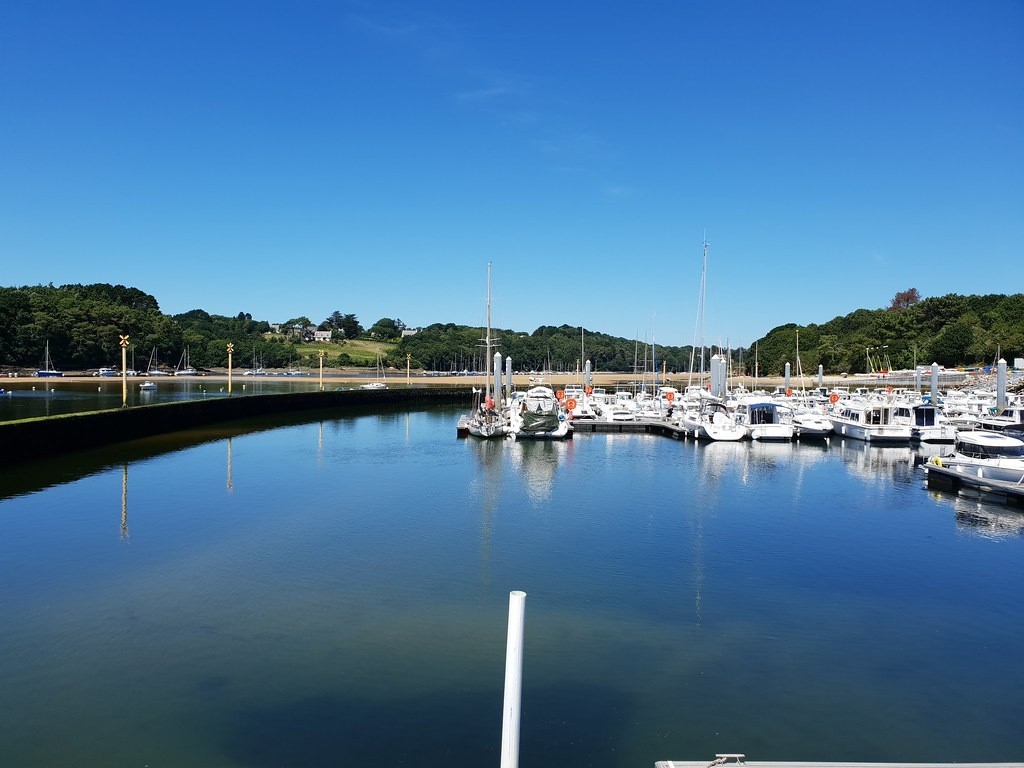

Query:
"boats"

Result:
[[92, 365, 139, 379]]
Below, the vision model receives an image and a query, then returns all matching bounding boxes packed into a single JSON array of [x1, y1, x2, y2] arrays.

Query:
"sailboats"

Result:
[[243, 347, 266, 376], [37, 340, 63, 377], [174, 345, 198, 376], [430, 223, 1022, 505], [146, 345, 168, 376]]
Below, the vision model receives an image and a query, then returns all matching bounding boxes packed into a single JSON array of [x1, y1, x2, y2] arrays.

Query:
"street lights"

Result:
[[118, 334, 131, 408], [226, 342, 234, 397], [405, 352, 411, 387], [318, 349, 325, 391]]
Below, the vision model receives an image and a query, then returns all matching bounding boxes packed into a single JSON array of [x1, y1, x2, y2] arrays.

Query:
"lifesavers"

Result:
[[788, 389, 792, 396], [556, 390, 564, 399], [566, 398, 576, 409], [666, 392, 674, 400], [585, 386, 591, 393], [933, 457, 943, 467], [888, 387, 891, 391], [830, 394, 839, 402]]
